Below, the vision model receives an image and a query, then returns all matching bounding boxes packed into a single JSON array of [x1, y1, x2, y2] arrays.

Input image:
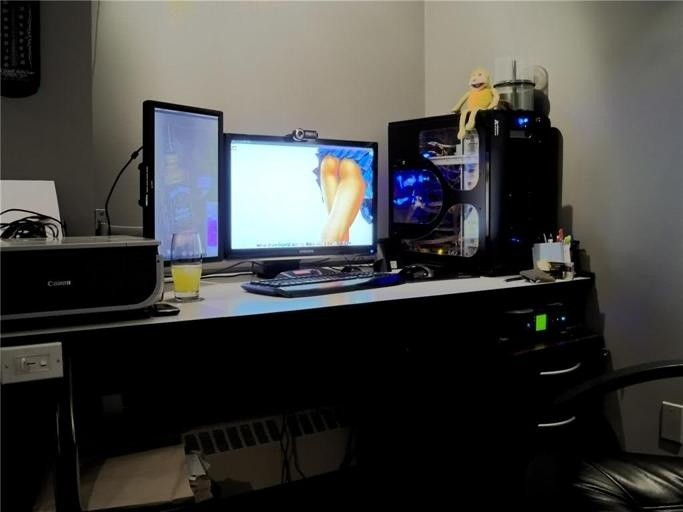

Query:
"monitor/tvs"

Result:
[[221, 129, 379, 280], [141, 98, 224, 264]]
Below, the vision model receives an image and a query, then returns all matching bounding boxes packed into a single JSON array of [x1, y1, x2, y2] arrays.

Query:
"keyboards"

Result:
[[242, 265, 407, 298]]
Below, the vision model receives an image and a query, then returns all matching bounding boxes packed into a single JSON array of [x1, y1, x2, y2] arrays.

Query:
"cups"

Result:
[[171, 232, 203, 302]]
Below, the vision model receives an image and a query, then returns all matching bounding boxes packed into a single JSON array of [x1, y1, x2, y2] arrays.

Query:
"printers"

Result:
[[0, 236, 163, 333]]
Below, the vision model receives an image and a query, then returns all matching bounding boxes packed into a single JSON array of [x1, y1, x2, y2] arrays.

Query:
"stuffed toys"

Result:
[[452, 67, 501, 142]]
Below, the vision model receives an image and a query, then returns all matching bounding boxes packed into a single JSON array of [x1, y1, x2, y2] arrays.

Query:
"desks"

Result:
[[0, 260, 597, 511]]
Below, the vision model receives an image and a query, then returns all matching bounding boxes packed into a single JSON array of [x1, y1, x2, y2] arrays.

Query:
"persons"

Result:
[[313, 144, 374, 246]]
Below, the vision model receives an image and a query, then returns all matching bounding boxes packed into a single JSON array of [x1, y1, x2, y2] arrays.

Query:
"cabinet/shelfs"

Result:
[[497, 317, 604, 450]]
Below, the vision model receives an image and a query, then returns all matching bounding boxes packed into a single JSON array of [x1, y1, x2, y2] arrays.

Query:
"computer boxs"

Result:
[[388, 110, 562, 275]]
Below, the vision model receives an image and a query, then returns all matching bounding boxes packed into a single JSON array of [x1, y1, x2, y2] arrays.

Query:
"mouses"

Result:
[[403, 265, 435, 279]]
